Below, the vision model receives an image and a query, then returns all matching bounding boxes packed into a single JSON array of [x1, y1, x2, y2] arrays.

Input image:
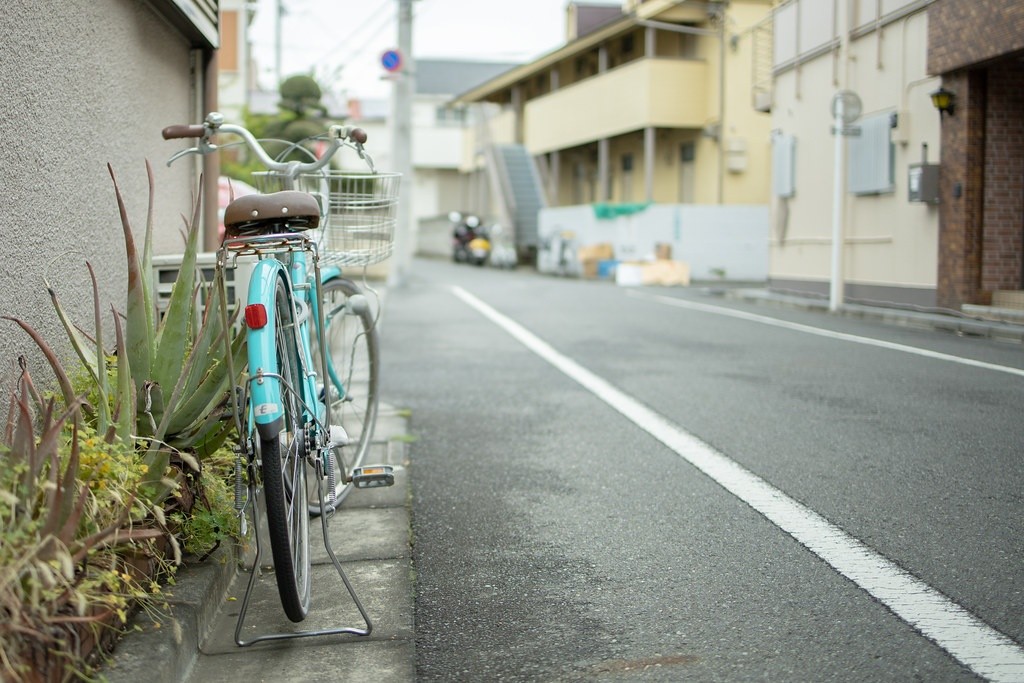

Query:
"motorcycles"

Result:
[[450, 211, 492, 265]]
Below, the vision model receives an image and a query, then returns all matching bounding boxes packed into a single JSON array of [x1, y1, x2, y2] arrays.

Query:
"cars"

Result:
[[218, 176, 264, 251]]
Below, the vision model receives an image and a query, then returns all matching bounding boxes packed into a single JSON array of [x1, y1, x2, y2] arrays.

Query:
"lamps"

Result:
[[930, 88, 956, 120]]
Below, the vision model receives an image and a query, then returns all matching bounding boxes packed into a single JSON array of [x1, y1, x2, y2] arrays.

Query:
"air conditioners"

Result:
[[150, 250, 257, 419]]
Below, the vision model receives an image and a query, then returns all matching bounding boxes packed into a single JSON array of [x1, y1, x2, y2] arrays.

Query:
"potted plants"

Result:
[[0, 156, 246, 683]]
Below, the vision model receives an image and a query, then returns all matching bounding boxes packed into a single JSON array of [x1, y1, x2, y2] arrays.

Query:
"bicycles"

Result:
[[162, 111, 404, 648]]
